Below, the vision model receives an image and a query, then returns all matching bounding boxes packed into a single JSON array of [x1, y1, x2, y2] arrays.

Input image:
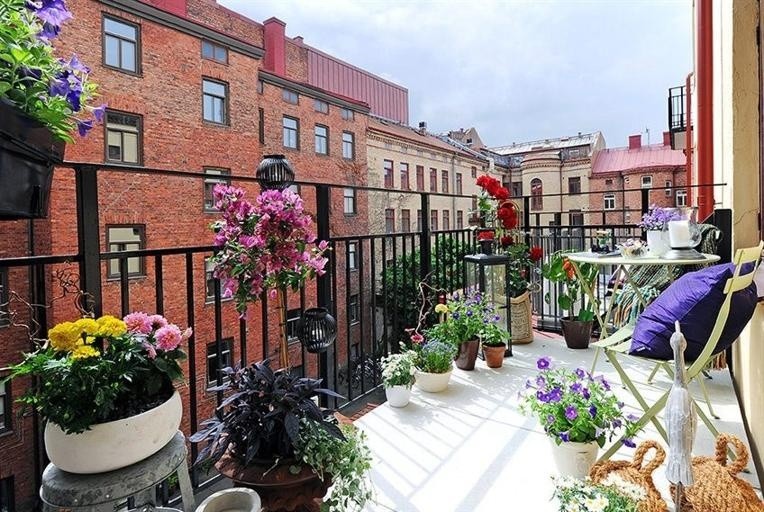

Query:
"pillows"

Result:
[[629, 261, 759, 366]]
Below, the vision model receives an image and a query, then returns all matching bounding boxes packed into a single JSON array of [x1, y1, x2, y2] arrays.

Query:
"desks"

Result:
[[569, 252, 722, 389]]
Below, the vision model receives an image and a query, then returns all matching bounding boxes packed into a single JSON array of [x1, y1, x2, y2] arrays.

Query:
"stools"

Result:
[[39, 430, 196, 512]]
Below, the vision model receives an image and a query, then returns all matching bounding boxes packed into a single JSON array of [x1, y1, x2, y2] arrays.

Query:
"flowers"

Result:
[[374, 350, 417, 390], [405, 303, 460, 360], [0, 311, 195, 437], [1, 1, 110, 145], [480, 304, 513, 345], [446, 286, 488, 341], [636, 203, 688, 231], [464, 174, 544, 297], [516, 356, 647, 451], [207, 181, 335, 322], [541, 248, 602, 323], [548, 472, 650, 512]]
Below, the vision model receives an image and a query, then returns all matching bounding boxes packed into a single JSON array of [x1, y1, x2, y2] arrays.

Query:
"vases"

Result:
[[647, 231, 671, 253], [481, 342, 506, 368], [559, 316, 591, 349], [455, 334, 480, 371], [385, 384, 410, 407], [548, 431, 599, 478], [0, 99, 67, 223], [43, 389, 185, 474]]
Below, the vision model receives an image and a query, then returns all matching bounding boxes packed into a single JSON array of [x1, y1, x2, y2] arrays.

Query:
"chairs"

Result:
[[605, 225, 724, 386], [588, 240, 764, 471]]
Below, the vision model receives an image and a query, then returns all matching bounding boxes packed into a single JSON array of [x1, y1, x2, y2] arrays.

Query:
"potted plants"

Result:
[[398, 340, 453, 393], [187, 358, 376, 512]]
